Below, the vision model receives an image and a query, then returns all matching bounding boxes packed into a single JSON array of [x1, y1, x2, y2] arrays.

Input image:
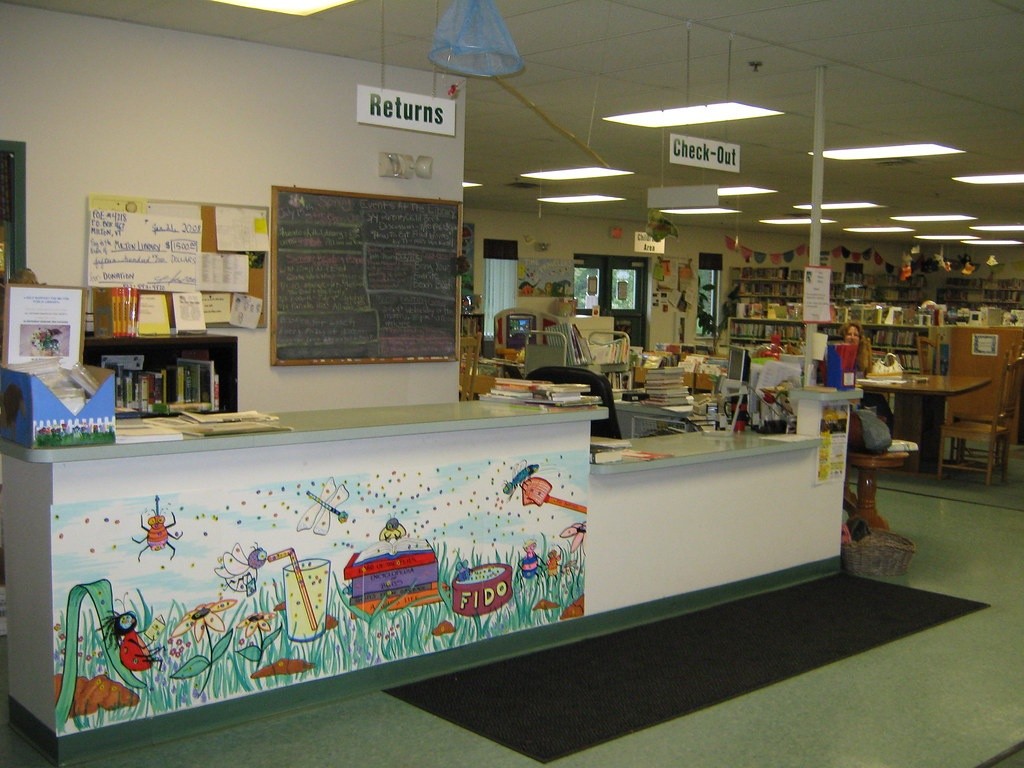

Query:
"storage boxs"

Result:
[[1, 365, 115, 449]]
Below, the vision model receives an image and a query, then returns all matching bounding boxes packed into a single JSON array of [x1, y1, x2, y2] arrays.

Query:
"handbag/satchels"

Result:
[[872, 353, 903, 377]]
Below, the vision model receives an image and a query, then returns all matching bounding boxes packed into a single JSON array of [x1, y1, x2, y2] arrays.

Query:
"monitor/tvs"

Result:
[[727, 346, 751, 420]]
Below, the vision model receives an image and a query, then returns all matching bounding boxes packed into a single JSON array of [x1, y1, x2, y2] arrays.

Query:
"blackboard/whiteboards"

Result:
[[84, 195, 268, 332], [271, 186, 462, 365]]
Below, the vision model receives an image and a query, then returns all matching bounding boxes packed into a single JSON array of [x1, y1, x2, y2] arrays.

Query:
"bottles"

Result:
[[764, 335, 785, 360]]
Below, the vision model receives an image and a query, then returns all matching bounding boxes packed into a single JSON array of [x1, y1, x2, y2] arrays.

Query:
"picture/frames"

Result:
[[3, 284, 89, 366]]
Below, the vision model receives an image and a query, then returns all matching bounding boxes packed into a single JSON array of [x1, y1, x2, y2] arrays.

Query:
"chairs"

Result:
[[916, 337, 940, 375], [524, 366, 622, 440], [937, 350, 1024, 485], [460, 332, 482, 402]]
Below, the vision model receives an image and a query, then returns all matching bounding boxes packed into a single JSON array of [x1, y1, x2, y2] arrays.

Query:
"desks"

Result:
[[858, 375, 992, 479], [847, 452, 910, 530]]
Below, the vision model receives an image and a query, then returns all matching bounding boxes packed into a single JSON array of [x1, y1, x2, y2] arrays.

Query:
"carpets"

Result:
[[382, 572, 992, 763]]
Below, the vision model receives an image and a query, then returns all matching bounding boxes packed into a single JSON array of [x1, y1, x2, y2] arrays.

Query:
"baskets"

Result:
[[841, 527, 916, 577]]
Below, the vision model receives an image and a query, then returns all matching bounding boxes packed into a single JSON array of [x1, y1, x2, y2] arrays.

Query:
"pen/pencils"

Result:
[[217, 418, 241, 423]]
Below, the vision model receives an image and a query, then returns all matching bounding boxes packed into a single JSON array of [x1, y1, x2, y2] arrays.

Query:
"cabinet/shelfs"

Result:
[[831, 272, 924, 304], [730, 266, 806, 304], [86, 333, 239, 421], [524, 330, 632, 390], [726, 317, 930, 374]]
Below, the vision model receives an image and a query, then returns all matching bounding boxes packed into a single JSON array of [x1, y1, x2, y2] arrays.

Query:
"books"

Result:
[[651, 342, 713, 356], [105, 359, 220, 412], [644, 366, 691, 407], [872, 350, 920, 370], [91, 286, 170, 338], [734, 322, 800, 340], [738, 267, 920, 306], [482, 377, 604, 407], [944, 277, 1024, 310], [641, 351, 673, 369], [603, 369, 632, 390], [590, 444, 623, 464], [545, 322, 628, 365], [820, 327, 841, 337], [678, 353, 729, 391], [172, 292, 207, 335], [116, 427, 183, 445], [872, 330, 919, 347]]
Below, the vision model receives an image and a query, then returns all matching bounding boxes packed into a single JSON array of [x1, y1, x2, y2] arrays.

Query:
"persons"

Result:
[[839, 323, 895, 437]]
